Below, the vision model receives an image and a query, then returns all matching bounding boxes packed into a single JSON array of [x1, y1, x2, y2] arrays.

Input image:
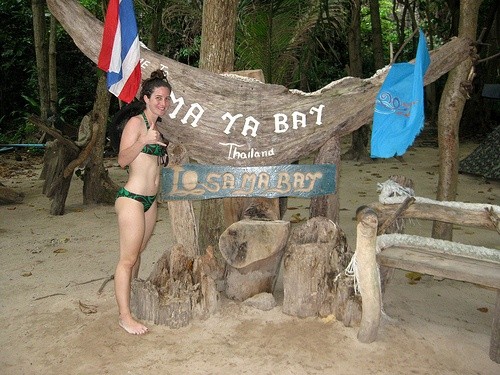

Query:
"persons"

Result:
[[114, 70, 172, 335], [75, 109, 94, 181]]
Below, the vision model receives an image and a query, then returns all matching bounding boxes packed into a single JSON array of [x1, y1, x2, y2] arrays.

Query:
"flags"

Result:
[[370, 28, 432, 158], [97, 0, 141, 103]]
